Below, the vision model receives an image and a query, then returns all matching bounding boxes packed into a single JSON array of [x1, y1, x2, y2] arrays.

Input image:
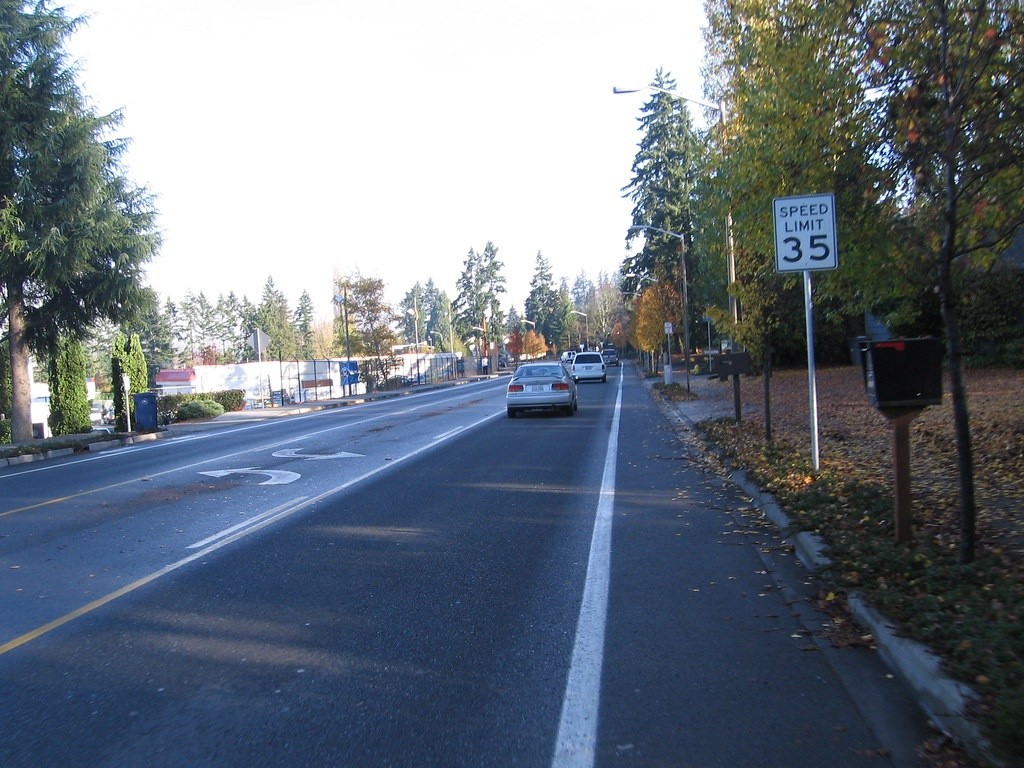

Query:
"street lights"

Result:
[[613, 82, 739, 354], [629, 224, 692, 372], [569, 310, 589, 351], [520, 319, 535, 335], [430, 329, 443, 344]]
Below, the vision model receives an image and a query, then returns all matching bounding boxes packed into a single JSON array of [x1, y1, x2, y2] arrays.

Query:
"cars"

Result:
[[30, 394, 50, 415], [505, 361, 579, 419], [601, 348, 619, 367], [99, 399, 115, 425], [560, 350, 577, 364], [571, 351, 607, 384]]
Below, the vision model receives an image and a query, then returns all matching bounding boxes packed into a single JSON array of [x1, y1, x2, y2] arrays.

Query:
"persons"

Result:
[[481, 357, 489, 375]]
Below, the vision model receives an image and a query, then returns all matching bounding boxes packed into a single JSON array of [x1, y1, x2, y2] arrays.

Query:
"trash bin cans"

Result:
[[133, 392, 157, 431]]
